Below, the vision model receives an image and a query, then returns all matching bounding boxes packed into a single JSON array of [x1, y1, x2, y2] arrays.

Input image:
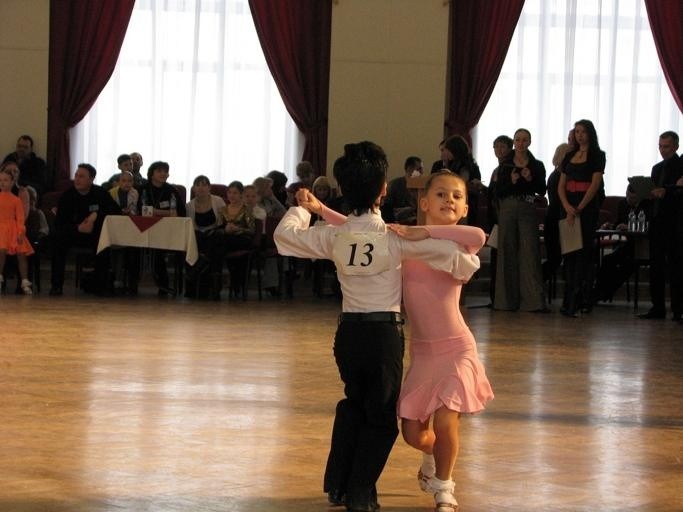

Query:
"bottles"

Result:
[[628, 208, 636, 232], [637, 209, 646, 233], [140, 189, 148, 207]]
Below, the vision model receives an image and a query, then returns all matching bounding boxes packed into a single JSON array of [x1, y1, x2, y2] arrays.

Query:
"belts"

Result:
[[505, 195, 542, 204], [339, 311, 406, 325]]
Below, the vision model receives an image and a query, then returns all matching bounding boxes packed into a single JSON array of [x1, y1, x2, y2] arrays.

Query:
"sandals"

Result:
[[435, 489, 459, 512], [21, 282, 33, 294], [327, 492, 346, 506], [418, 464, 436, 491], [345, 498, 382, 512]]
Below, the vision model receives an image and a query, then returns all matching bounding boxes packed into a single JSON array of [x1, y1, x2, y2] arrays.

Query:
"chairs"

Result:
[[34, 179, 652, 311]]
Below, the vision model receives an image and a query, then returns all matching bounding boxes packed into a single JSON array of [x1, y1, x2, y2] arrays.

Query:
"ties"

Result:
[[653, 161, 664, 216]]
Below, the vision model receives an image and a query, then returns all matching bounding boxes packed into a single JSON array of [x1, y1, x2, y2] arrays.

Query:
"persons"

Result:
[[612, 130, 682, 323], [298, 170, 495, 512], [385, 135, 482, 229], [2, 132, 372, 303], [271, 140, 480, 512], [485, 128, 546, 311], [546, 120, 606, 316]]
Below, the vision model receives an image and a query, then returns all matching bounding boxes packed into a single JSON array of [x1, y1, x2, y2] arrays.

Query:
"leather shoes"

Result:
[[674, 314, 683, 323], [637, 312, 665, 318]]
